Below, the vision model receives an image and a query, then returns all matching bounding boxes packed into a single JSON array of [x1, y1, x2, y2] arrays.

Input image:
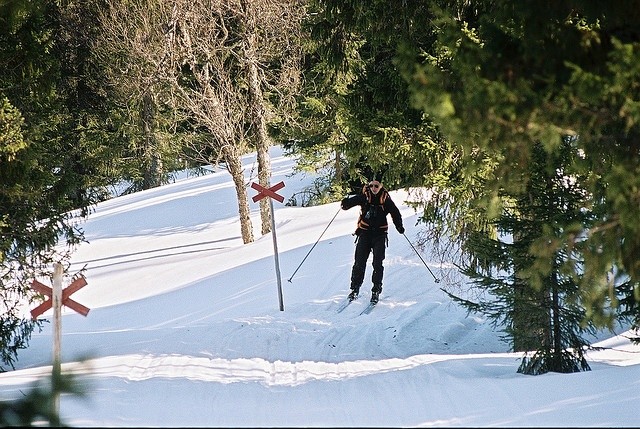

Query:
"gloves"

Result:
[[395, 219, 403, 234], [342, 198, 349, 208]]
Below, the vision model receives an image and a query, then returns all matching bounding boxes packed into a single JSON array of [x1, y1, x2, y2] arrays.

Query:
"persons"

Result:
[[341, 174, 405, 305]]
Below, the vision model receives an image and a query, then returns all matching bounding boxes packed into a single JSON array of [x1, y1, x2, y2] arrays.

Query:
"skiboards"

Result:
[[336, 297, 376, 316]]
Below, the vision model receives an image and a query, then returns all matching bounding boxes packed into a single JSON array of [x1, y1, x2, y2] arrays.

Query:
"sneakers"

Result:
[[370, 292, 378, 304], [348, 288, 359, 301]]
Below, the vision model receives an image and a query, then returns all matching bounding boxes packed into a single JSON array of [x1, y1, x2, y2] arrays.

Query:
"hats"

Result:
[[369, 173, 382, 183]]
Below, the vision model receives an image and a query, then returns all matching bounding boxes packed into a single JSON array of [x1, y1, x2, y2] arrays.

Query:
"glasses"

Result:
[[368, 183, 381, 189]]
